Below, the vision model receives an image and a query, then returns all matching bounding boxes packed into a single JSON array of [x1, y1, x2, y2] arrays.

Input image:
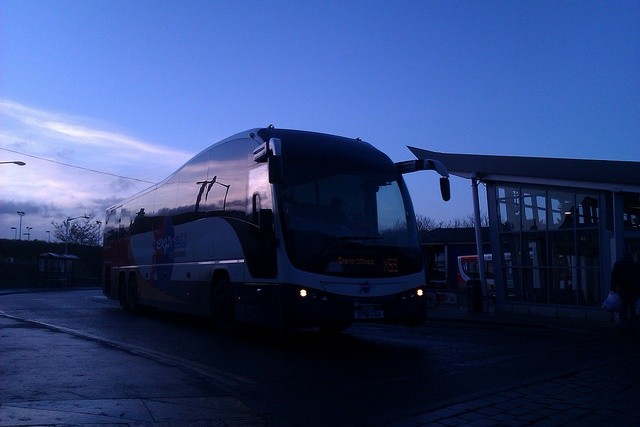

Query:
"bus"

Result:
[[457, 250, 534, 298], [101, 126, 450, 328]]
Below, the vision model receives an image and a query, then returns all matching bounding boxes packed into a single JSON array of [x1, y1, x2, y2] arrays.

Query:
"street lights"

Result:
[[65, 216, 90, 254], [16, 209, 25, 239], [11, 226, 17, 239], [0, 160, 26, 167], [46, 230, 50, 243], [27, 226, 32, 240]]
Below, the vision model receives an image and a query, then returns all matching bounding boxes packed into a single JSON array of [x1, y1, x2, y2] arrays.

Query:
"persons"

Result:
[[611, 246, 640, 329]]
